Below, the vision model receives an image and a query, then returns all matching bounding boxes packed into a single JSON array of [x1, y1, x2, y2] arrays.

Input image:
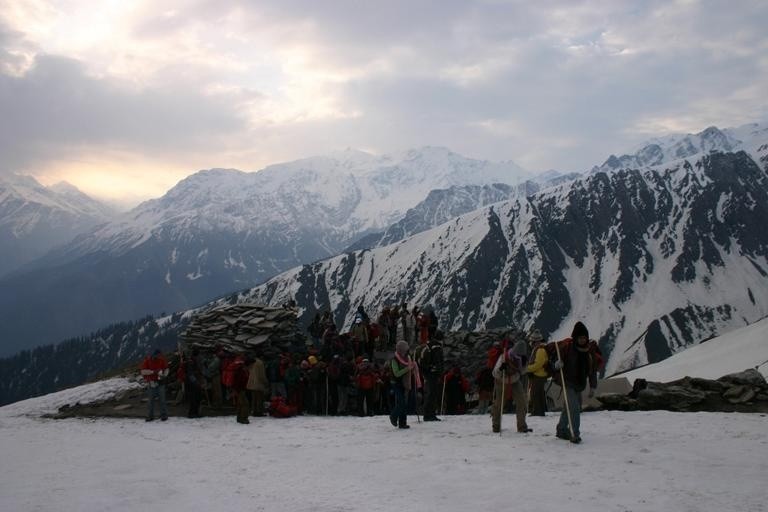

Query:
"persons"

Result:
[[138, 304, 547, 433], [551, 322, 598, 443]]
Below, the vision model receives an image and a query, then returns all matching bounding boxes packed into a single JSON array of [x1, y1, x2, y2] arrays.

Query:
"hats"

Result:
[[308, 356, 317, 365], [355, 318, 362, 323], [396, 340, 409, 354], [571, 322, 588, 338], [513, 340, 527, 355], [529, 329, 543, 341]]
